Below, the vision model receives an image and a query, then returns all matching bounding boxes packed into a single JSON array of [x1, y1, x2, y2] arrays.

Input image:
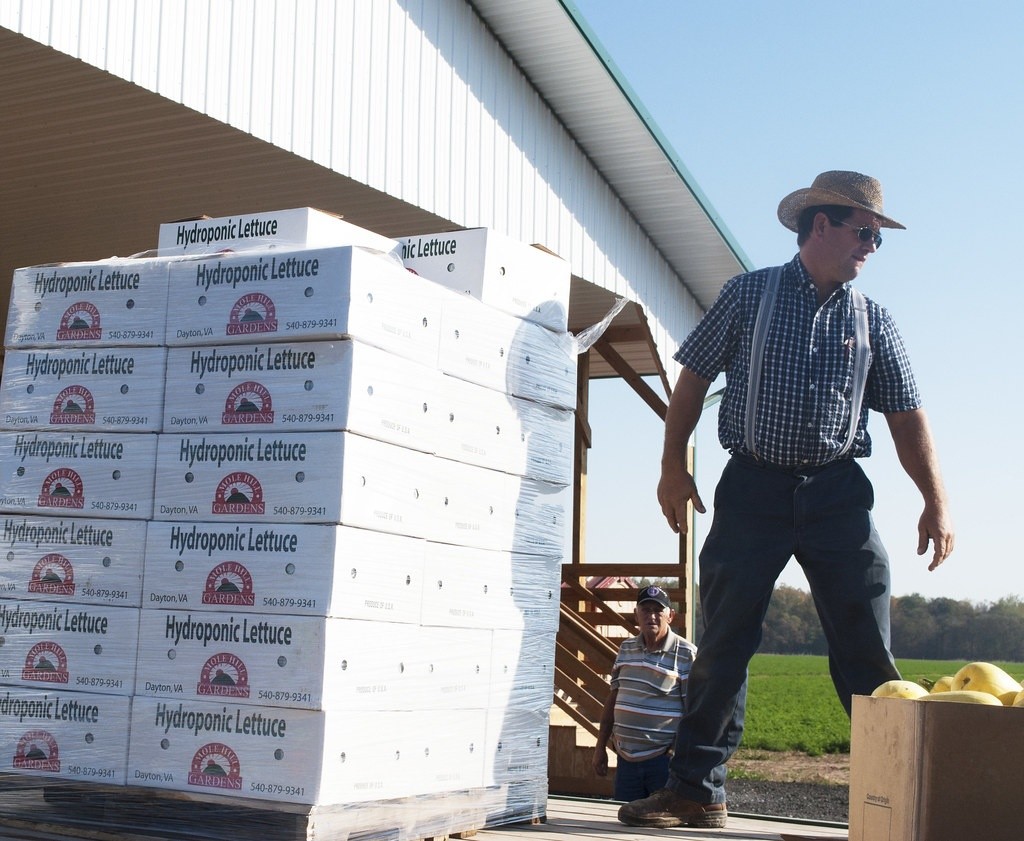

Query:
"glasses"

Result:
[[828, 216, 882, 250]]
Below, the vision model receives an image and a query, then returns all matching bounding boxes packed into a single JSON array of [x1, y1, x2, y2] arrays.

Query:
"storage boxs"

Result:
[[848, 693, 1023, 841], [0, 208, 578, 806]]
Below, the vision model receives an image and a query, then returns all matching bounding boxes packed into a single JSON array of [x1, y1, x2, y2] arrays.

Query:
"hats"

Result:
[[776, 171, 906, 233], [637, 585, 671, 609]]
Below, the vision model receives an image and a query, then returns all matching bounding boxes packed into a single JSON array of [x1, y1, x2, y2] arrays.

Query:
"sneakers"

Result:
[[618, 786, 727, 828]]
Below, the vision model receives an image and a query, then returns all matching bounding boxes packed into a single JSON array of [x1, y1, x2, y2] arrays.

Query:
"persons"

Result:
[[616, 169, 956, 829], [591, 584, 700, 803]]
